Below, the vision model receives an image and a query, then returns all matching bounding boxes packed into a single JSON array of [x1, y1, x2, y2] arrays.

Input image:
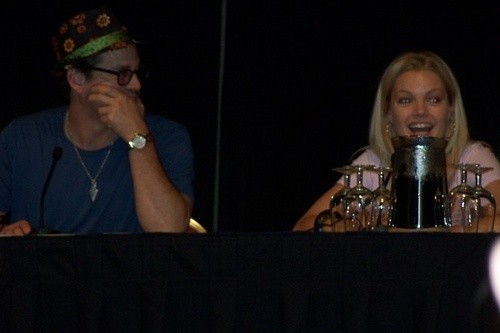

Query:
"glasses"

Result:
[[89, 63, 145, 86]]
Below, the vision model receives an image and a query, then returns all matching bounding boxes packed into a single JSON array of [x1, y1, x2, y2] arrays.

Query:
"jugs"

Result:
[[390, 136, 446, 229]]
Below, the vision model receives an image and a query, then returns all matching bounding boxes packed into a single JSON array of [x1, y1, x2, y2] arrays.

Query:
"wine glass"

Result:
[[329, 165, 395, 232], [444, 163, 496, 232]]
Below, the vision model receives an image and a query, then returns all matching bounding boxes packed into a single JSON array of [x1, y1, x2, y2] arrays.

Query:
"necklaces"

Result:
[[65, 110, 118, 204]]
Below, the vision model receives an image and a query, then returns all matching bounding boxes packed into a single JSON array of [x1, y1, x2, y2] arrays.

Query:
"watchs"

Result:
[[125, 133, 154, 151]]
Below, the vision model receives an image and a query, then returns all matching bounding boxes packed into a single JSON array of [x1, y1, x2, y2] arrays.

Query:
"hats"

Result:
[[50, 7, 146, 63]]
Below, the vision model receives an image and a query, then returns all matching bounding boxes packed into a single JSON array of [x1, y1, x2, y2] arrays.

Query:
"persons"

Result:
[[291, 51, 500, 233], [0, 10, 196, 235]]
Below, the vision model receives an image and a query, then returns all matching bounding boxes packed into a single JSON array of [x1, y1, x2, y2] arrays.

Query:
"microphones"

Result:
[[32, 146, 63, 234], [364, 154, 394, 208]]
